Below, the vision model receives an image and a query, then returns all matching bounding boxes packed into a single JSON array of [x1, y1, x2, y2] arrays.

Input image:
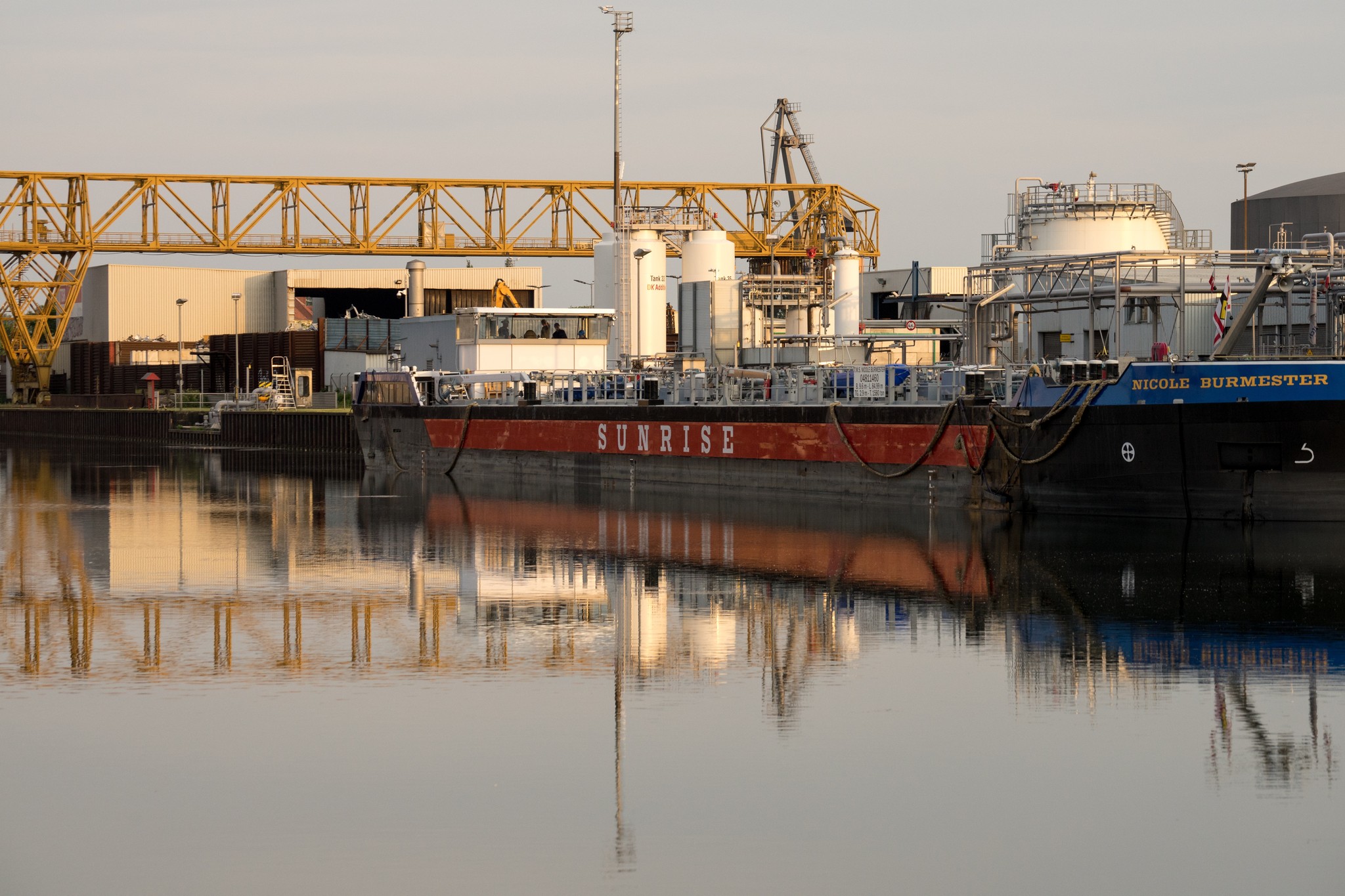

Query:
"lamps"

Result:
[[877, 278, 887, 285], [891, 291, 899, 298], [1168, 353, 1180, 374], [474, 313, 480, 320], [397, 280, 402, 284], [596, 313, 605, 318], [486, 312, 495, 318], [420, 395, 426, 402], [546, 380, 552, 383], [1302, 275, 1311, 286], [627, 383, 634, 388], [609, 315, 618, 321]]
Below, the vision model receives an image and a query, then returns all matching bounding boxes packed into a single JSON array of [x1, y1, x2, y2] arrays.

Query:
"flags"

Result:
[[1308, 277, 1316, 345], [1209, 269, 1215, 291], [1322, 273, 1331, 293], [1213, 277, 1231, 346]]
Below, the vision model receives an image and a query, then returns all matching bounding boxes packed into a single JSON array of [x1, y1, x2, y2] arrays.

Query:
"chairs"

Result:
[[524, 329, 537, 338]]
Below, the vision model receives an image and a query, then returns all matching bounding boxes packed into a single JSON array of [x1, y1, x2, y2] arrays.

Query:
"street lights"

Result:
[[633, 248, 653, 360], [666, 275, 682, 300], [1236, 162, 1258, 268], [231, 293, 242, 400], [176, 299, 189, 411], [526, 285, 552, 308], [708, 268, 720, 280], [573, 280, 594, 308], [766, 233, 780, 368]]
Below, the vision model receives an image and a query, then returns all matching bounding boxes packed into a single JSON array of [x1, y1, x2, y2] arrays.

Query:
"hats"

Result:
[[578, 330, 585, 336]]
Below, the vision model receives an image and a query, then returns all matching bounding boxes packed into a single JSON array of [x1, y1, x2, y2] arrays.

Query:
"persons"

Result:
[[541, 319, 550, 339], [498, 320, 511, 339], [552, 323, 568, 338], [578, 330, 587, 339]]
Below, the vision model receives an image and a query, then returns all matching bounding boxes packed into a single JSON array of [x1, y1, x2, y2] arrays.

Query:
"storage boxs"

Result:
[[31, 219, 52, 237], [287, 238, 338, 243]]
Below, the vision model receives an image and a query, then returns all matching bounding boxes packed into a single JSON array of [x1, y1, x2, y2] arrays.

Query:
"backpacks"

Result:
[[524, 330, 537, 338]]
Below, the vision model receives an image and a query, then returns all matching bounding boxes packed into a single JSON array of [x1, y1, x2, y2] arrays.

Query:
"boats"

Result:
[[350, 344, 1005, 502], [990, 337, 1344, 479], [348, 464, 1004, 643]]
